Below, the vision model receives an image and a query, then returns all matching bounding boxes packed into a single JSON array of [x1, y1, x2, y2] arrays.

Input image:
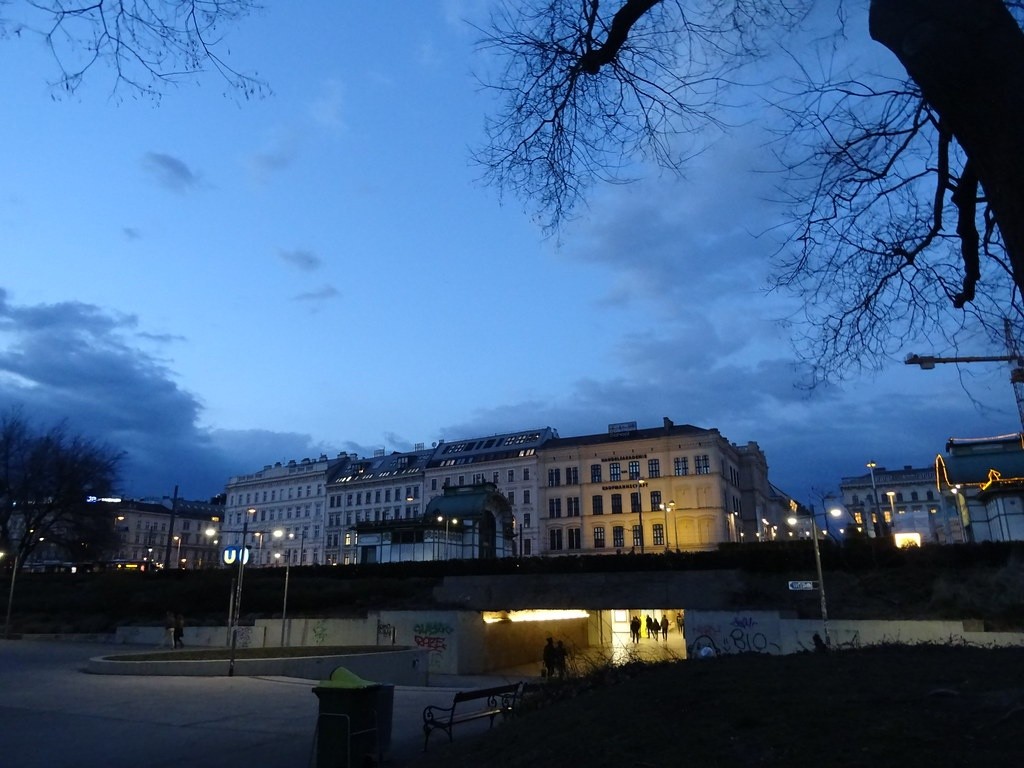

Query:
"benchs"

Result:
[[423, 681, 523, 752], [502, 680, 566, 721]]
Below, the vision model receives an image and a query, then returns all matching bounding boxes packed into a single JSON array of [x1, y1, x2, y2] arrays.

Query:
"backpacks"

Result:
[[680, 619, 683, 626]]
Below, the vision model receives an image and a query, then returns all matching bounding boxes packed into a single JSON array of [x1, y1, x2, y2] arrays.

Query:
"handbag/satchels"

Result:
[[541, 669, 546, 677]]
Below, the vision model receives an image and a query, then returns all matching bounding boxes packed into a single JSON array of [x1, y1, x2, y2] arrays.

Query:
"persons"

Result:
[[542, 636, 569, 678], [158, 609, 185, 650], [626, 611, 685, 644]]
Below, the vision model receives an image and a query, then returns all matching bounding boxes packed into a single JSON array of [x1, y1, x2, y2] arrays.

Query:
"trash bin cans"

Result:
[[311, 683, 395, 768]]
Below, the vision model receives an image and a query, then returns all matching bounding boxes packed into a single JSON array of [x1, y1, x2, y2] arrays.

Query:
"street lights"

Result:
[[638, 478, 645, 554], [659, 503, 671, 549], [289, 530, 303, 566], [787, 505, 842, 646], [669, 500, 680, 553], [205, 523, 283, 677], [275, 553, 290, 647], [255, 533, 263, 566], [867, 461, 884, 536], [174, 536, 181, 569], [437, 516, 458, 561]]
